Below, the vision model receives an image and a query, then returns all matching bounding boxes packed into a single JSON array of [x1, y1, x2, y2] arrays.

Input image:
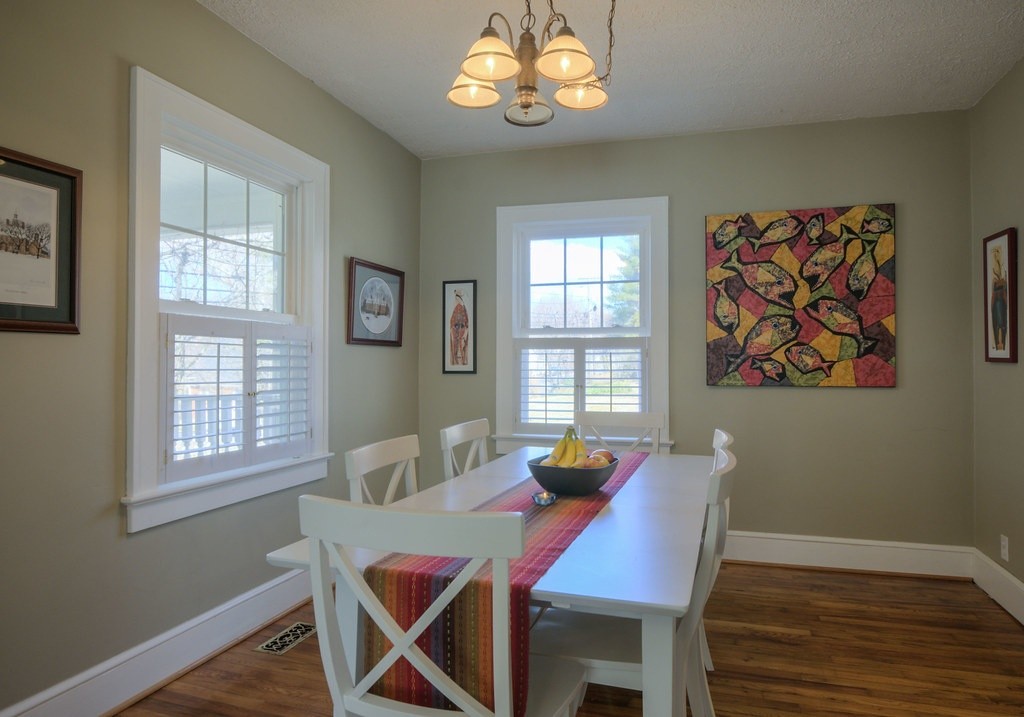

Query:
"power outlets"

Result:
[[1001, 534, 1008, 561]]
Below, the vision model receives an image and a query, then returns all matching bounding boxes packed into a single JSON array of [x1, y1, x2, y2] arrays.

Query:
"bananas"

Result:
[[538, 425, 586, 468]]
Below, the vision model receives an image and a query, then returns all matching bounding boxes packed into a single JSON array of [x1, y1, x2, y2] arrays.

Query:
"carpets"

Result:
[[251, 620, 317, 655]]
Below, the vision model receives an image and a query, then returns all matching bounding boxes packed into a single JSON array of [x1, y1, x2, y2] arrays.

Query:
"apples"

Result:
[[584, 449, 615, 469]]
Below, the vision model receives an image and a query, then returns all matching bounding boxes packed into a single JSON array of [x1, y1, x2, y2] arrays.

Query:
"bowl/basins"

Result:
[[526, 453, 620, 498]]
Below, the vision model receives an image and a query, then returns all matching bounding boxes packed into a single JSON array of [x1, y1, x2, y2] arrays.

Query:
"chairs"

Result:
[[344, 433, 422, 504], [575, 409, 665, 453], [527, 428, 738, 717], [439, 417, 490, 480], [298, 494, 589, 717]]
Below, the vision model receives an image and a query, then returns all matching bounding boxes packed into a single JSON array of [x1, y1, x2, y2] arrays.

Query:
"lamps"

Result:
[[445, 0, 617, 126]]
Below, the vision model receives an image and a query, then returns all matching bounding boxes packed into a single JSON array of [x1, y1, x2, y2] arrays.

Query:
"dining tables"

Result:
[[265, 444, 715, 716]]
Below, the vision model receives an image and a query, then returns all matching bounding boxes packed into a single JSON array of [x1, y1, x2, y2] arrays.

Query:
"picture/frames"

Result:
[[983, 227, 1018, 362], [443, 279, 477, 374], [347, 255, 405, 346], [0, 145, 84, 334]]
